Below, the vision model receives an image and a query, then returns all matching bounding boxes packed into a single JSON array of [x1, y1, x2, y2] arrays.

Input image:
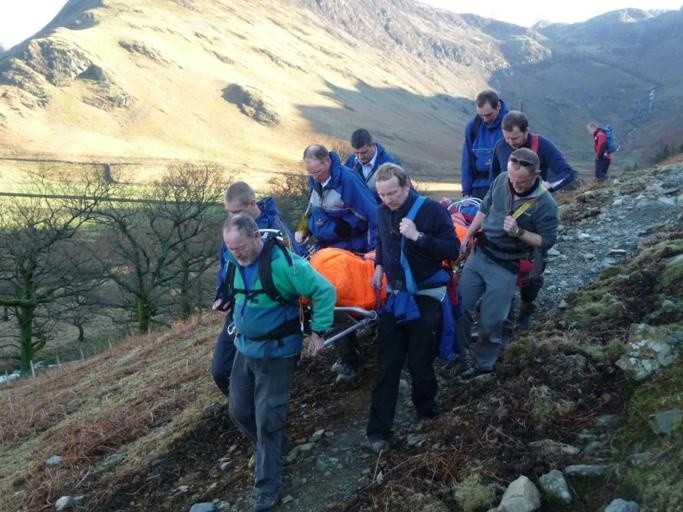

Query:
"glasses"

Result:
[[511, 154, 534, 167]]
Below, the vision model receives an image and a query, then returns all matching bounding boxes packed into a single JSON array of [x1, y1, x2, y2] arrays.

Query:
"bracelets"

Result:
[[514, 227, 527, 238]]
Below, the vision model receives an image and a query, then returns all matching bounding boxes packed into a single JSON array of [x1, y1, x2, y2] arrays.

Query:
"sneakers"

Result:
[[331, 349, 367, 386], [438, 351, 494, 379], [366, 438, 392, 452], [253, 487, 279, 510]]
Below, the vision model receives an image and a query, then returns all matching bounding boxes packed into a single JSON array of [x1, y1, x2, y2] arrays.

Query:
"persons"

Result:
[[490, 110, 575, 320], [367, 161, 460, 454], [209, 178, 306, 399], [288, 145, 377, 385], [347, 130, 409, 345], [460, 91, 510, 200], [585, 121, 612, 182], [453, 146, 557, 387], [221, 211, 338, 511]]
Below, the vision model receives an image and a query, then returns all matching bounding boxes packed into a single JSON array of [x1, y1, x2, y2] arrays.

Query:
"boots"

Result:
[[520, 302, 537, 330]]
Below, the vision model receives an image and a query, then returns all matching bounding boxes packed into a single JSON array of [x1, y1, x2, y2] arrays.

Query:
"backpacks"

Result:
[[225, 229, 289, 305], [605, 124, 618, 153]]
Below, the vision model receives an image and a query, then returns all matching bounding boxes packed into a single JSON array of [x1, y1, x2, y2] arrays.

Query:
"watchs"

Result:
[[414, 232, 423, 241]]
